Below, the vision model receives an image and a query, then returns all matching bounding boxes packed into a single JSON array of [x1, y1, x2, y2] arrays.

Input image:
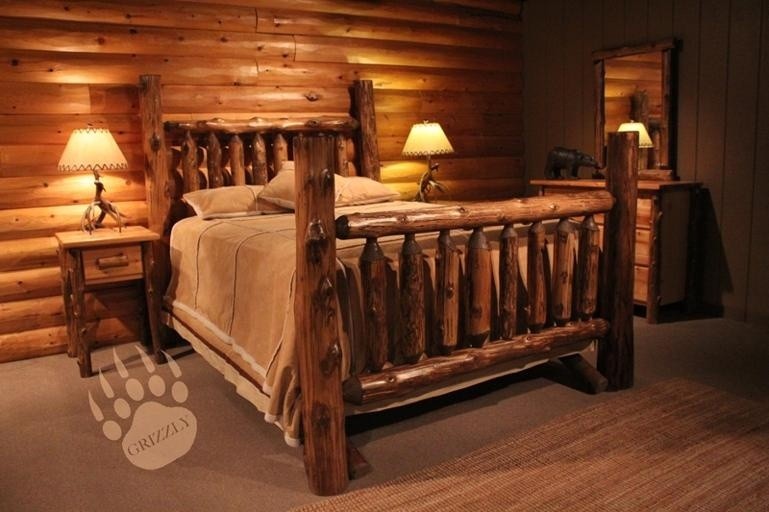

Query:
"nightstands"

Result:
[[55, 223, 167, 376]]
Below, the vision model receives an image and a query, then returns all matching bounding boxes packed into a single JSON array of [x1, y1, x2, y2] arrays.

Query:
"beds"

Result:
[[137, 73, 639, 495]]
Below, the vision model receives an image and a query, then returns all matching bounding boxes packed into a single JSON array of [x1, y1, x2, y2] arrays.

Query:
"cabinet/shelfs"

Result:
[[531, 179, 702, 325]]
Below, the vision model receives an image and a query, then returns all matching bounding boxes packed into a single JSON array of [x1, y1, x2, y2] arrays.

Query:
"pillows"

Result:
[[339, 175, 401, 209], [259, 160, 348, 213], [181, 184, 290, 221]]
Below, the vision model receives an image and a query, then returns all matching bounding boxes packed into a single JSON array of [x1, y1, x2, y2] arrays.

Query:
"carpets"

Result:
[[283, 371, 769, 512]]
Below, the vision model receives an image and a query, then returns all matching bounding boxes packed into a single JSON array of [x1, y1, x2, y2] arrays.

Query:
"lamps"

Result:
[[616, 122, 653, 153], [401, 118, 454, 199], [57, 123, 135, 232]]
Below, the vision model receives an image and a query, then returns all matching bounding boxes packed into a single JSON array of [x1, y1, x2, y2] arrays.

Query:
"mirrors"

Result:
[[591, 35, 681, 180]]
